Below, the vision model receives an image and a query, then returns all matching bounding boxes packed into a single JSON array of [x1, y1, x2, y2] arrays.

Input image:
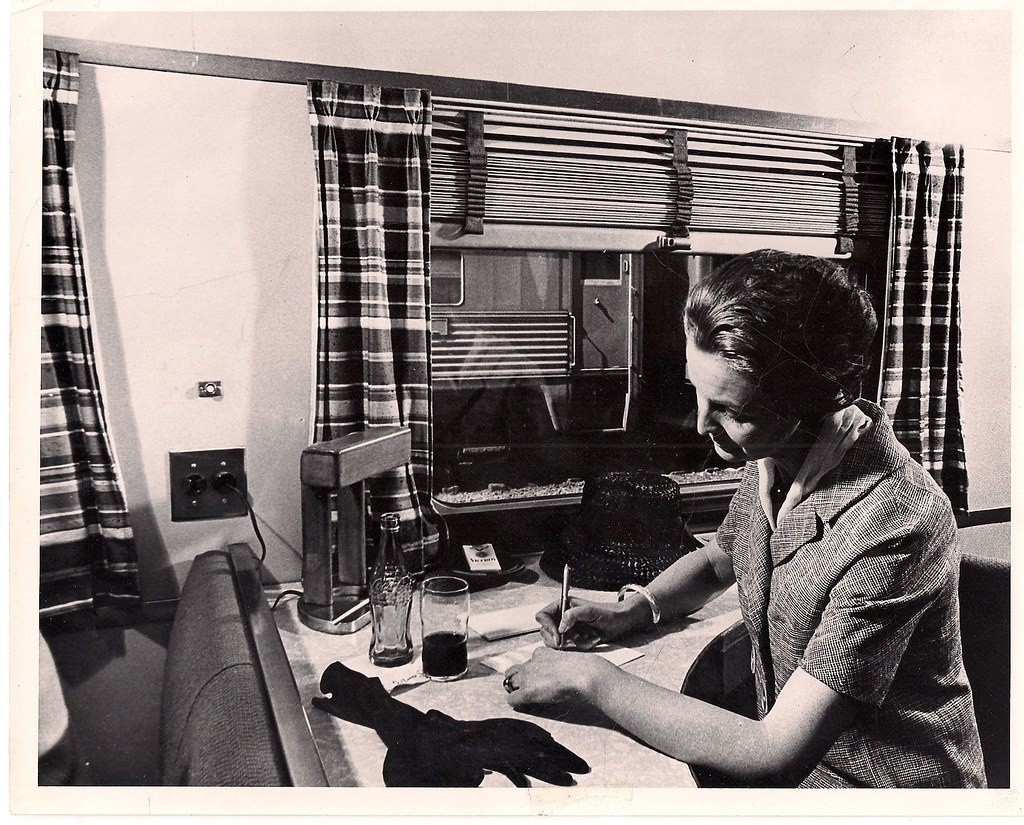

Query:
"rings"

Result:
[[504, 674, 515, 694]]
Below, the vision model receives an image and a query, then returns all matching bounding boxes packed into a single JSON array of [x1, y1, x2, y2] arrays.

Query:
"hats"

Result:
[[539, 472, 705, 592]]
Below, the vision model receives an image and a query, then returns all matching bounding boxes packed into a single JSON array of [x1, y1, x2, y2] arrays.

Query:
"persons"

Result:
[[504, 249, 989, 788]]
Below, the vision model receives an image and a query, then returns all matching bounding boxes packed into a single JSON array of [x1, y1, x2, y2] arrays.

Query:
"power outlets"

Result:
[[169, 448, 248, 522]]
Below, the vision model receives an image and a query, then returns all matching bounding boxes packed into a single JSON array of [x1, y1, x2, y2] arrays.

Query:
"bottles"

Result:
[[368, 512, 413, 668]]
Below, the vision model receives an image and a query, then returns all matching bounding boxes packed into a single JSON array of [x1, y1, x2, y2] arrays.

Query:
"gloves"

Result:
[[312, 661, 590, 787]]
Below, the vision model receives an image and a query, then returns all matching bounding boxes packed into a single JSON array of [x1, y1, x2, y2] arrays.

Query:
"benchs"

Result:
[[45, 542, 283, 787]]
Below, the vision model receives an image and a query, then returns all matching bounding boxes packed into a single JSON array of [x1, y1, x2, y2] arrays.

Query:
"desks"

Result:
[[260, 530, 755, 788]]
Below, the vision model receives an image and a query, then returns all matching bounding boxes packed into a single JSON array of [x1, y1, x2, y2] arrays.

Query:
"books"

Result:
[[466, 602, 554, 642]]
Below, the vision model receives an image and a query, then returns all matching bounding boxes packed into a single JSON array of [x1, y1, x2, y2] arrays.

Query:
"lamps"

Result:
[[296, 425, 411, 634]]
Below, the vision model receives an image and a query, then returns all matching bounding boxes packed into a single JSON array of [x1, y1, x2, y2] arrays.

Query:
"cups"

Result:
[[419, 576, 470, 682]]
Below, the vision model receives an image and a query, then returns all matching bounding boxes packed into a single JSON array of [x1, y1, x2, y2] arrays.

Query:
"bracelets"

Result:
[[615, 582, 663, 633]]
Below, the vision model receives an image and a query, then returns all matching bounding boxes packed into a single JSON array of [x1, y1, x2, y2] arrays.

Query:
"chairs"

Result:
[[951, 554, 1011, 789]]
